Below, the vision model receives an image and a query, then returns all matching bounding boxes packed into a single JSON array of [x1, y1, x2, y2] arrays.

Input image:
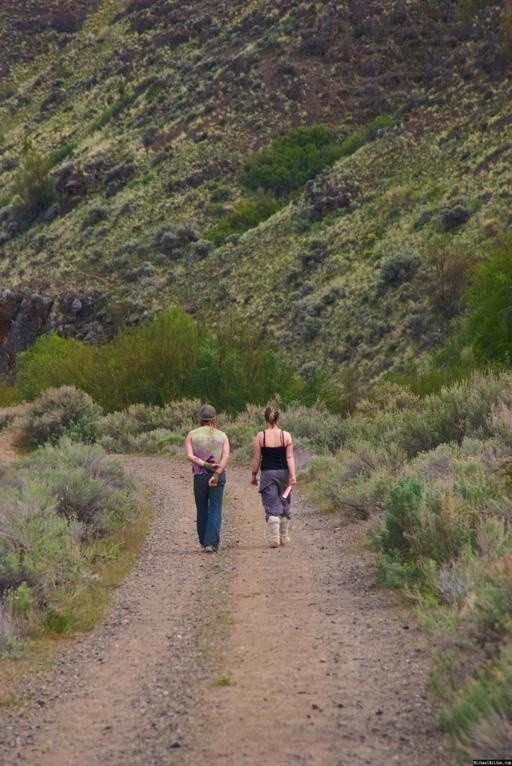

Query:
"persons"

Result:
[[250, 406, 297, 548], [186, 404, 231, 552]]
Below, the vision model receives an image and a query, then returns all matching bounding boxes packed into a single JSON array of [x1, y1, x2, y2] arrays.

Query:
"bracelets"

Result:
[[203, 462, 210, 469], [252, 471, 257, 475], [213, 472, 220, 479]]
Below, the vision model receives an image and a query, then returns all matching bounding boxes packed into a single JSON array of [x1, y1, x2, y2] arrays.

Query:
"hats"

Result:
[[200, 405, 216, 420]]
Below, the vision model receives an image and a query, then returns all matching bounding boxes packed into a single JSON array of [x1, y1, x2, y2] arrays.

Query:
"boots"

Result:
[[266, 515, 280, 548], [281, 517, 290, 545]]
